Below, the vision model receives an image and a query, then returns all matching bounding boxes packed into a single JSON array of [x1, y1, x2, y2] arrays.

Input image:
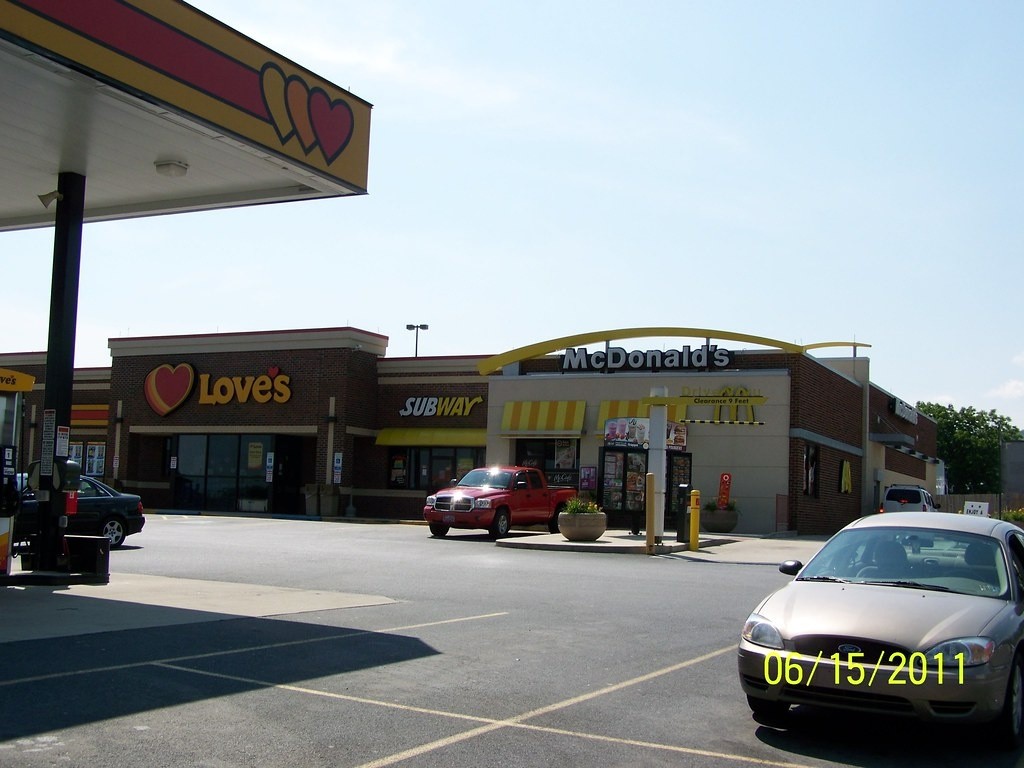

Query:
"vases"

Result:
[[558, 512, 606, 542], [700, 508, 738, 533]]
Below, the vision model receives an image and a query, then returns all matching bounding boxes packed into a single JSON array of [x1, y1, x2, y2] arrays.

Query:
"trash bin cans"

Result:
[[305, 484, 320, 516], [320, 485, 340, 516]]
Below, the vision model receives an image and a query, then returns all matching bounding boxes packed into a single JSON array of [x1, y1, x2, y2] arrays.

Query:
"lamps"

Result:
[[407, 324, 428, 357], [154, 161, 190, 178]]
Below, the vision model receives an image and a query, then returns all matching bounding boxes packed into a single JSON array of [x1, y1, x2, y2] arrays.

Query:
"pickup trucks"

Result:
[[423, 465, 578, 540]]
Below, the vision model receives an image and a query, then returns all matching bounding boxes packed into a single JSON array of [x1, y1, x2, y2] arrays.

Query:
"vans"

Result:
[[879, 483, 941, 513]]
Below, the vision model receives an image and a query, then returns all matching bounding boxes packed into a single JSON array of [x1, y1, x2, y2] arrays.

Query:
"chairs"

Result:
[[856, 541, 921, 578], [959, 543, 999, 587]]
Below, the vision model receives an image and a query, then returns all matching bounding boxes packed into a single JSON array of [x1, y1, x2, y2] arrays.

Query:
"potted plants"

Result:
[[237, 471, 269, 512], [991, 507, 1024, 530]]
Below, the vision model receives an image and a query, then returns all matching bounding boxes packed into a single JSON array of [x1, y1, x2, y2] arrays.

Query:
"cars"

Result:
[[737, 511, 1024, 752], [16, 476, 145, 548]]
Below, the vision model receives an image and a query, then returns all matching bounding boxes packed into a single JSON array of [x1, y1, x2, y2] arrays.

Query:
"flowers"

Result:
[[558, 497, 606, 513], [700, 496, 738, 509]]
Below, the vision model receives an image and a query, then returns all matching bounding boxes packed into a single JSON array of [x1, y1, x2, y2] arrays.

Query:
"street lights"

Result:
[[406, 324, 429, 357]]
